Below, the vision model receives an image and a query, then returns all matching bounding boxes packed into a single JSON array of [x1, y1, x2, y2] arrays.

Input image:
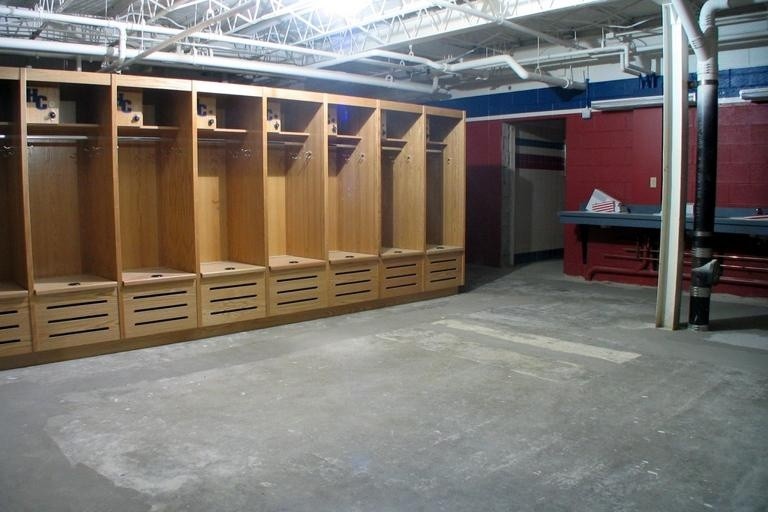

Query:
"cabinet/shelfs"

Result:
[[0, 64, 467, 370]]
